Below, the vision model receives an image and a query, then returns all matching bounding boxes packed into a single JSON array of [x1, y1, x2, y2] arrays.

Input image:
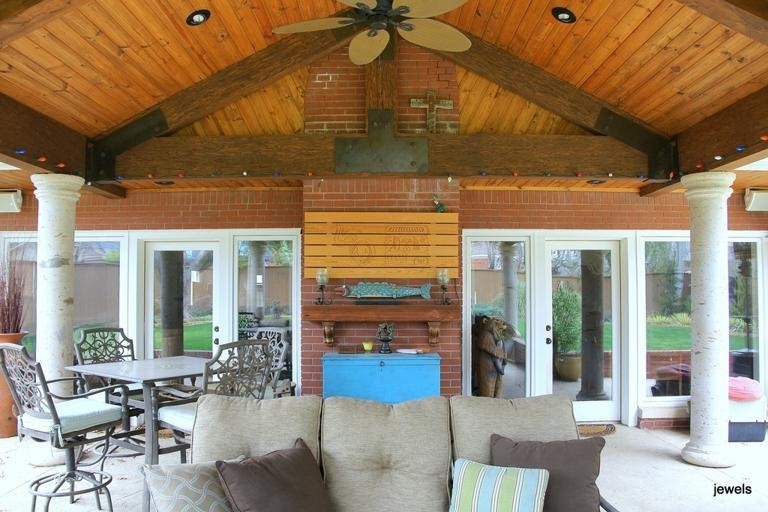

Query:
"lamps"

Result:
[[438, 268, 451, 305], [313, 269, 334, 305], [0, 188, 22, 214], [744, 187, 768, 212]]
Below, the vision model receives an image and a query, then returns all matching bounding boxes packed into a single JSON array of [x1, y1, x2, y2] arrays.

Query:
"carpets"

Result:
[[578, 423, 616, 437]]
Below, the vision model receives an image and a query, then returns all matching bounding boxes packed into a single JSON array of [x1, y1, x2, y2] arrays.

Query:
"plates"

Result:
[[397, 348, 420, 353]]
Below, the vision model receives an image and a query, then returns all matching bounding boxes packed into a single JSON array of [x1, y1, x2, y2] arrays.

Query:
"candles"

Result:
[[553, 282, 582, 382]]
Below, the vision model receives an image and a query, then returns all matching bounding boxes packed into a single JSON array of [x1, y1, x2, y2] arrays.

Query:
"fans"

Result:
[[272, 0, 472, 66]]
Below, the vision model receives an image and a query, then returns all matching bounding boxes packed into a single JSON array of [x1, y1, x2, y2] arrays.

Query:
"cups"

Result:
[[363, 342, 373, 353], [428, 321, 441, 345], [322, 321, 336, 342]]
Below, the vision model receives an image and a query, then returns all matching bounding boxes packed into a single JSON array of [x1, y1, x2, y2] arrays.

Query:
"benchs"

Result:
[[141, 394, 619, 512]]
